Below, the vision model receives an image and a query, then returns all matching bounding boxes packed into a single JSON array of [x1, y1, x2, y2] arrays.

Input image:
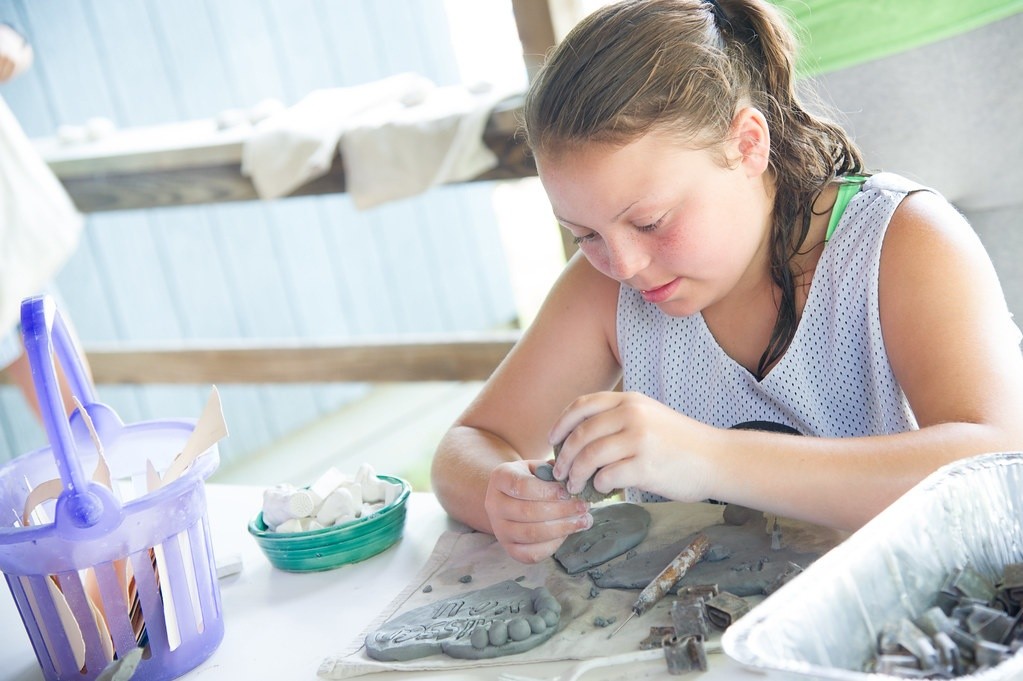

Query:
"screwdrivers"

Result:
[[606, 531, 713, 639]]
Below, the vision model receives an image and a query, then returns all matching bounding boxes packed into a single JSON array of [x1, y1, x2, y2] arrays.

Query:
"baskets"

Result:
[[0, 295, 225, 681]]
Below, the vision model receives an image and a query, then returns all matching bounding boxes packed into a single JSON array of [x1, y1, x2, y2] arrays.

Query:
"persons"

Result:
[[430, 0, 1023, 564], [0, 24, 87, 430]]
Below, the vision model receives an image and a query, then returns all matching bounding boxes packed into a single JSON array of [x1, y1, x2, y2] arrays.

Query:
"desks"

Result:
[[0, 484, 889, 681]]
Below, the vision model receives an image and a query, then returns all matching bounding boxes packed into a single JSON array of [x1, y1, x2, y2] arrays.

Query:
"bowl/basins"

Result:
[[249, 476, 410, 572]]
